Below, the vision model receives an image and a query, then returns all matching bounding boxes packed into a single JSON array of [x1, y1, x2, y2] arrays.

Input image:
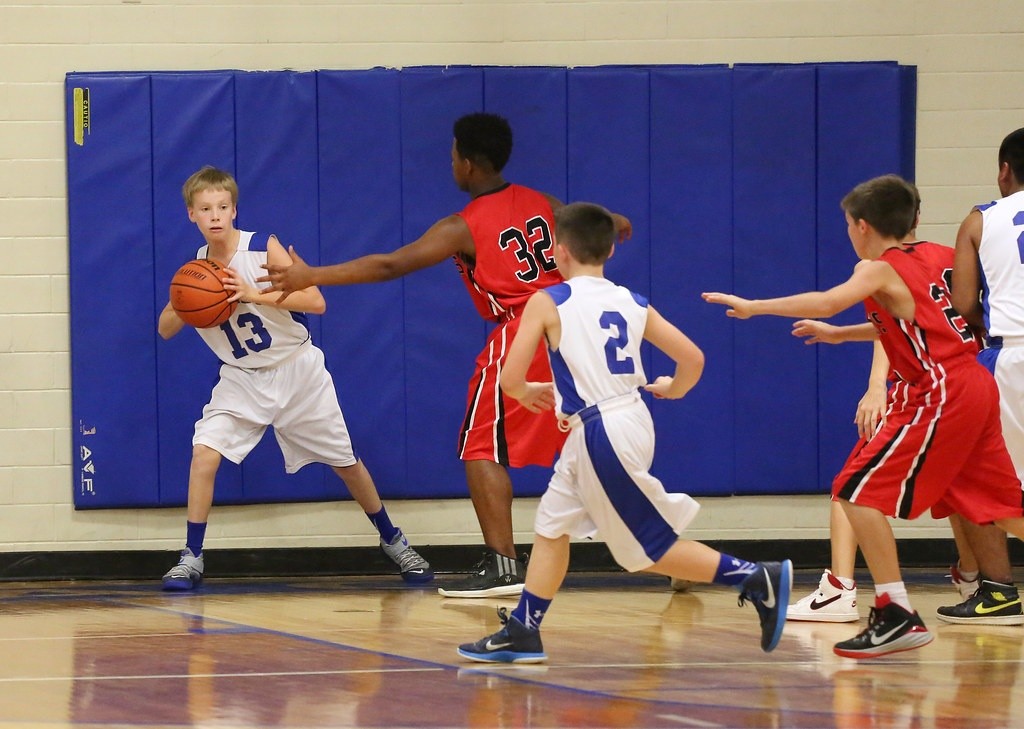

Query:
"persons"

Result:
[[935, 126, 1024, 625], [253, 110, 634, 598], [156, 165, 434, 590], [700, 173, 985, 658], [453, 200, 794, 665], [786, 182, 989, 625]]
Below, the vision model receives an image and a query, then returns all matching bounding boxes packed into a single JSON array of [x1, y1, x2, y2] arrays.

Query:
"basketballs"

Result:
[[170, 258, 239, 328]]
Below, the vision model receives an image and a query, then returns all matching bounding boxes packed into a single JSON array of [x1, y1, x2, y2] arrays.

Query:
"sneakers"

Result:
[[379, 527, 433, 581], [438, 548, 527, 598], [457, 628, 547, 666], [948, 558, 979, 603], [751, 559, 794, 653], [936, 580, 1023, 626], [163, 548, 206, 591], [833, 591, 935, 659], [785, 570, 859, 623]]
